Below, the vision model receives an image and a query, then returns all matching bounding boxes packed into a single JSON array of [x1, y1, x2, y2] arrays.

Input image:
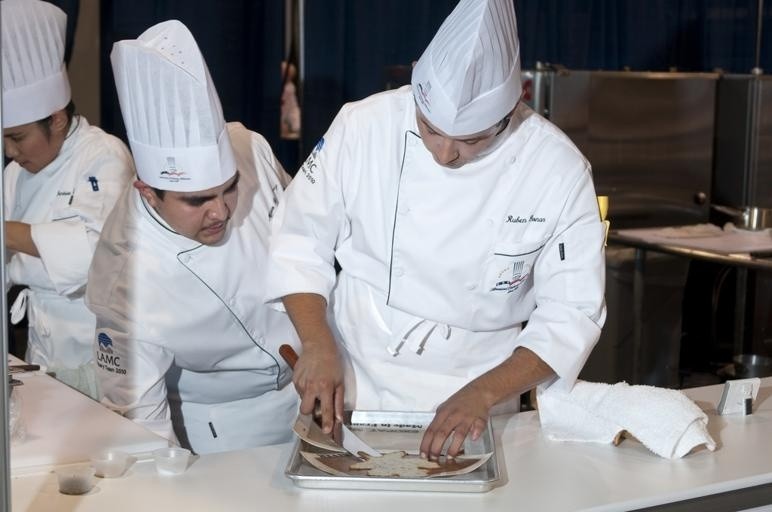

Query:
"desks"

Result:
[[5, 352, 771, 512], [606, 230, 771, 382]]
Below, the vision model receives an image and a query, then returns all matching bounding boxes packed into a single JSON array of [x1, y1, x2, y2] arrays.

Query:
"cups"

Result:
[[149, 446, 193, 478], [57, 448, 128, 495]]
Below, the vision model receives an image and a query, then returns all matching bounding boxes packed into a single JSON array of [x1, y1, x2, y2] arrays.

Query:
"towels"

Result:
[[536, 378, 716, 461]]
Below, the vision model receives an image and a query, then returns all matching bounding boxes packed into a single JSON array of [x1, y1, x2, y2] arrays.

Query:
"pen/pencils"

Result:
[[87, 176, 98, 190]]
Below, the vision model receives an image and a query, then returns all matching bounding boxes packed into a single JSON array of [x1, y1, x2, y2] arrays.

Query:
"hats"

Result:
[[109, 19, 239, 194], [0, 0, 74, 130]]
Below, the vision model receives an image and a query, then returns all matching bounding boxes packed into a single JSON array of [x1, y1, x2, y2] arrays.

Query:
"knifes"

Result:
[[280, 341, 382, 462]]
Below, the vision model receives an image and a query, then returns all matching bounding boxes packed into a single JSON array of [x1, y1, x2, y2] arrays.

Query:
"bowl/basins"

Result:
[[732, 354, 769, 376]]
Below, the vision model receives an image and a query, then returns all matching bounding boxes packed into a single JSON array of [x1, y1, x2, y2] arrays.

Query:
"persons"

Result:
[[258, 84, 613, 467], [82, 119, 314, 459], [1, 97, 141, 403]]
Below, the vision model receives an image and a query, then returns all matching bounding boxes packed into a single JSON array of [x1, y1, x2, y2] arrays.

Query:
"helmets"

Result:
[[410, 0, 524, 137]]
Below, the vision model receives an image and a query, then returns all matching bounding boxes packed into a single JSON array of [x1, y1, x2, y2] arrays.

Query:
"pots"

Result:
[[710, 200, 770, 231]]
